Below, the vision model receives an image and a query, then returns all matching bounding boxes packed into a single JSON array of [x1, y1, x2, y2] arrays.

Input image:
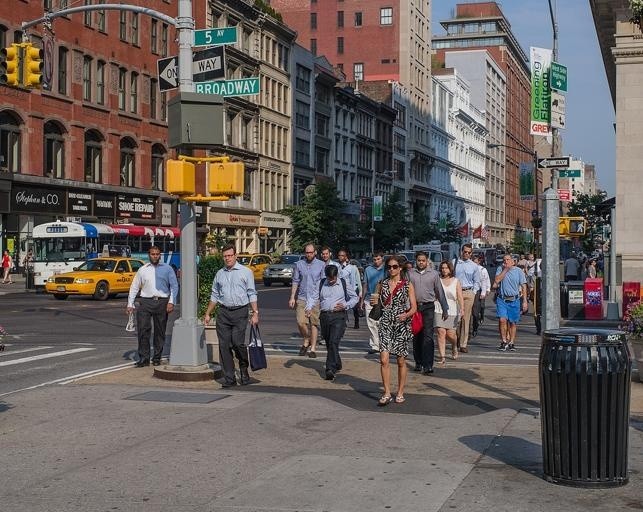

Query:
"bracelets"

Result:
[[254, 310, 259, 313]]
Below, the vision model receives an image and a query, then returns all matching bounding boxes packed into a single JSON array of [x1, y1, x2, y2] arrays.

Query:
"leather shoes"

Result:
[[222, 377, 248, 387], [415, 347, 468, 372], [326, 369, 334, 380], [368, 350, 377, 354], [135, 359, 150, 367], [472, 331, 478, 337], [153, 359, 160, 365]]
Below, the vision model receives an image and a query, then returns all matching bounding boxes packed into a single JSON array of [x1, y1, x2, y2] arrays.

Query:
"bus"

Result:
[[22, 220, 182, 288]]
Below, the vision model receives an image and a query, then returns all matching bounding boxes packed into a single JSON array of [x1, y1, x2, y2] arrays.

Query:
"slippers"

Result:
[[378, 396, 405, 405]]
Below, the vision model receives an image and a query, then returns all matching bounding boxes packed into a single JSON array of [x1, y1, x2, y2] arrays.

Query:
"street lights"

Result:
[[488, 141, 540, 260]]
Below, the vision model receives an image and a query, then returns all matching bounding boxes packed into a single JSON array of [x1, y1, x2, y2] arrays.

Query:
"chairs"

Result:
[[48, 252, 60, 261]]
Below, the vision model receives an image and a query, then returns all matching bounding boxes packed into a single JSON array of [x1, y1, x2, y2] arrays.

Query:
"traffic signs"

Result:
[[551, 64, 568, 89], [195, 78, 260, 96], [157, 46, 225, 93], [551, 92, 565, 112], [537, 158, 570, 168], [557, 189, 572, 202], [551, 113, 567, 128]]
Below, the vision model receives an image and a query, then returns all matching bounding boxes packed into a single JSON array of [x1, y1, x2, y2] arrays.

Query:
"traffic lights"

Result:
[[22, 42, 44, 92], [0, 42, 19, 87], [156, 158, 195, 196], [559, 217, 586, 237]]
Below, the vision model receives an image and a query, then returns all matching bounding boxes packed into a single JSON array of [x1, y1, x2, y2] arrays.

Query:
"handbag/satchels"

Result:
[[249, 324, 267, 371], [411, 311, 423, 334], [126, 313, 135, 331], [369, 304, 383, 320], [497, 295, 521, 302]]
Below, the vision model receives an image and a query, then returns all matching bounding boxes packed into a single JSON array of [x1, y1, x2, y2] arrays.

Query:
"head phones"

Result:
[[320, 248, 332, 259]]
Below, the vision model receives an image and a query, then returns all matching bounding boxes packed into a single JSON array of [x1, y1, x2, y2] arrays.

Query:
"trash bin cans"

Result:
[[27, 267, 34, 288], [539, 328, 631, 487]]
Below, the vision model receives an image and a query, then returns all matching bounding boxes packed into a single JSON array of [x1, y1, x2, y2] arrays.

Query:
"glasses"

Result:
[[306, 250, 315, 254], [224, 254, 234, 256], [387, 265, 400, 269]]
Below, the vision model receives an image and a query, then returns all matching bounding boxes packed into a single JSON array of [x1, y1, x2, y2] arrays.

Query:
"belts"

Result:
[[145, 297, 169, 300], [223, 305, 249, 311], [417, 302, 432, 305], [462, 288, 471, 290]]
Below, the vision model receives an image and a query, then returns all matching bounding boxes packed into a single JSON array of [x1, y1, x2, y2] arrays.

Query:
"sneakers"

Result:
[[299, 339, 326, 358], [497, 342, 517, 351]]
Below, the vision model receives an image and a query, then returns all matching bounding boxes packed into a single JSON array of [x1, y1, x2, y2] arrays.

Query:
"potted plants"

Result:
[[197, 253, 254, 365]]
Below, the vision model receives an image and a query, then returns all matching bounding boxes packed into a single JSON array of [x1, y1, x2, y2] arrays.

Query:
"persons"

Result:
[[26, 249, 34, 260], [368, 256, 419, 405], [404, 251, 450, 376], [449, 244, 480, 354], [287, 243, 324, 359], [0, 248, 14, 285], [317, 264, 361, 381], [203, 243, 261, 386], [315, 246, 606, 340], [125, 244, 180, 367], [359, 252, 387, 354], [492, 252, 530, 351], [433, 258, 467, 364]]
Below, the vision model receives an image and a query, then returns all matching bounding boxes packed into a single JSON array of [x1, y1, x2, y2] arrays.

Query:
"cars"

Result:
[[46, 255, 151, 301], [263, 254, 307, 287], [238, 254, 272, 283], [337, 238, 525, 279]]
